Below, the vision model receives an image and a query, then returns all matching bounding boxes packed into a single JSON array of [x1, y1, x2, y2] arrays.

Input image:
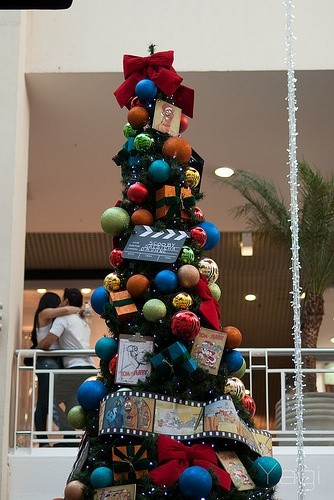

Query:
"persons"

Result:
[[35, 288, 97, 448], [31, 292, 86, 448]]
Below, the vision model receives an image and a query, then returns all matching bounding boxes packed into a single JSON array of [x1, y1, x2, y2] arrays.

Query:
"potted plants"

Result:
[[210, 158, 334, 446]]
